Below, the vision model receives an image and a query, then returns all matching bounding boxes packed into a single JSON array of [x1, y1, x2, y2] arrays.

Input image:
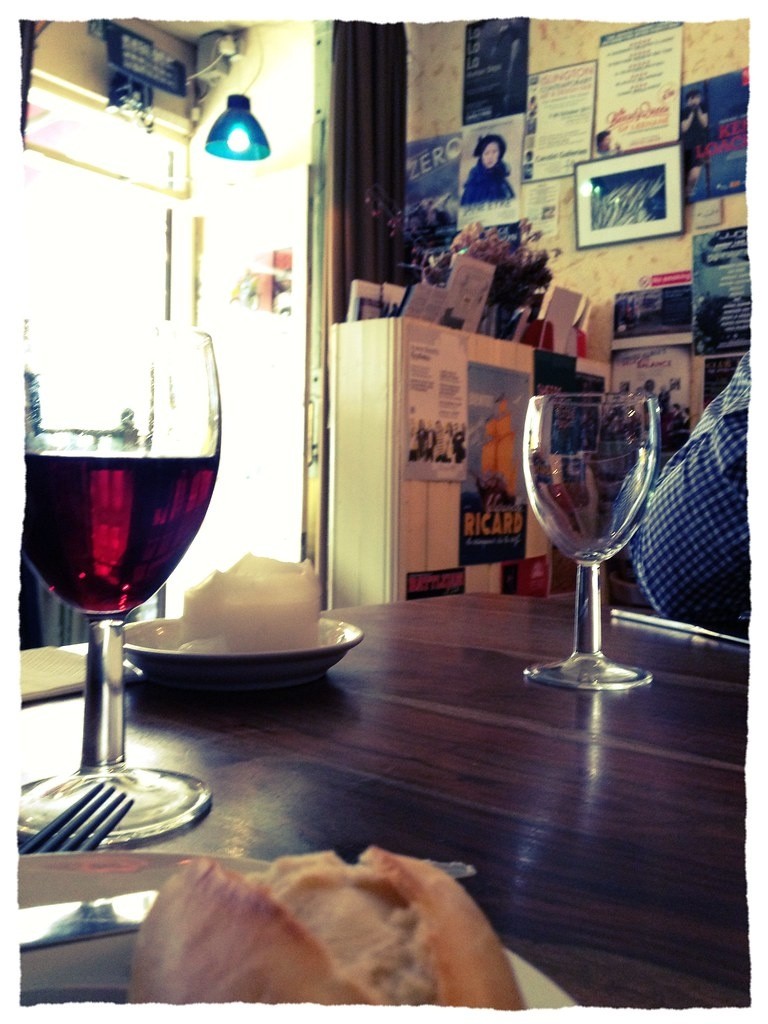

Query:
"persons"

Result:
[[596, 130, 620, 152], [618, 378, 691, 448], [461, 133, 515, 203], [626, 346, 749, 619], [680, 89, 708, 197], [409, 418, 467, 463]]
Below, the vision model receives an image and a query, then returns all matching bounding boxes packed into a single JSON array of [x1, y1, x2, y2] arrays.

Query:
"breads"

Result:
[[131, 845, 526, 1011]]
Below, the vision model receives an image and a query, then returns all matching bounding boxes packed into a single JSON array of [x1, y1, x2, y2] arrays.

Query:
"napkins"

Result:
[[20, 647, 138, 706]]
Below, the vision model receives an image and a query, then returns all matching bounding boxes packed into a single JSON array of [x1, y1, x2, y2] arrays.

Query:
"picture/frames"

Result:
[[573, 141, 683, 249]]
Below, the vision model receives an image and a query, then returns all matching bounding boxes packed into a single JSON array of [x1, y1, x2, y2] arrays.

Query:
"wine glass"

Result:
[[523, 394, 661, 690], [16, 312, 222, 844]]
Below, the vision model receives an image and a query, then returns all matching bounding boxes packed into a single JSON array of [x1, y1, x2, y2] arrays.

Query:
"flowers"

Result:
[[396, 218, 553, 303]]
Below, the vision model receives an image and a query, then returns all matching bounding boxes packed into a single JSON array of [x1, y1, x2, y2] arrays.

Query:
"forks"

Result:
[[19, 783, 134, 850]]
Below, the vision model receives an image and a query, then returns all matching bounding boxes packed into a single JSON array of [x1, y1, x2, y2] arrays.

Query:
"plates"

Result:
[[120, 616, 362, 682], [19, 851, 574, 1009]]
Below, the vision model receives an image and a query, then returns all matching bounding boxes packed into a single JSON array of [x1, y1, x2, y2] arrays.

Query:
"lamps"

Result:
[[205, 93, 269, 163]]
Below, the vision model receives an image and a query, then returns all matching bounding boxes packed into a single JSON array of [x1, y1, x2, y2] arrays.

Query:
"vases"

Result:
[[473, 302, 500, 338]]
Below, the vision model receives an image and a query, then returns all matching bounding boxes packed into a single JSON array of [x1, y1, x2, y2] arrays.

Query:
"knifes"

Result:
[[19, 890, 161, 949]]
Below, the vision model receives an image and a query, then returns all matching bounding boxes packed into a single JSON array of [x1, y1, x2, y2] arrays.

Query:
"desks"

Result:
[[20, 596, 752, 1007]]
